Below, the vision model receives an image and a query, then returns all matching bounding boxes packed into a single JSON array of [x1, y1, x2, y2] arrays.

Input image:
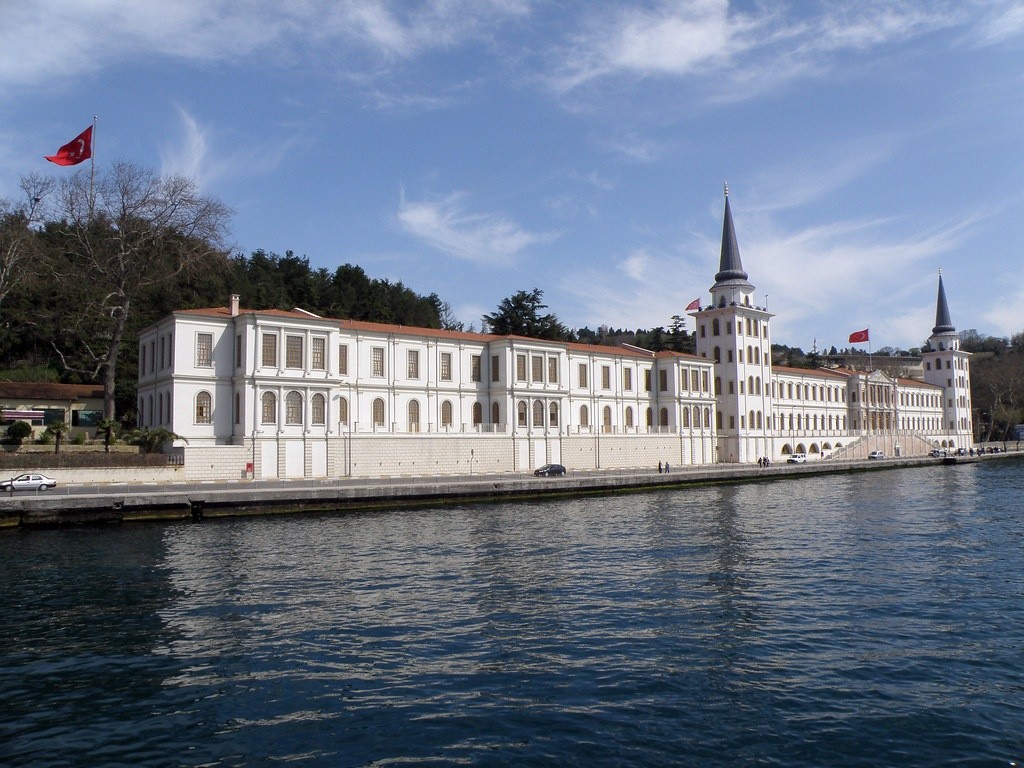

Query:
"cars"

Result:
[[928, 446, 1001, 458], [534, 464, 566, 477], [868, 450, 885, 459], [0, 474, 57, 492]]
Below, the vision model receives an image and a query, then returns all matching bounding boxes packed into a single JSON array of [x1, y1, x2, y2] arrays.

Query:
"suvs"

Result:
[[787, 453, 808, 464]]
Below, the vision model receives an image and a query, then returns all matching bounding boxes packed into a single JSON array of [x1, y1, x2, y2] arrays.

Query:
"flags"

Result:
[[849, 329, 869, 343], [43, 125, 93, 166], [685, 299, 700, 310]]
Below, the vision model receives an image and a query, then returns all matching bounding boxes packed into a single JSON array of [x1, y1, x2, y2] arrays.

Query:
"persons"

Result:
[[944, 446, 1007, 457], [659, 461, 670, 473], [758, 457, 770, 468]]
